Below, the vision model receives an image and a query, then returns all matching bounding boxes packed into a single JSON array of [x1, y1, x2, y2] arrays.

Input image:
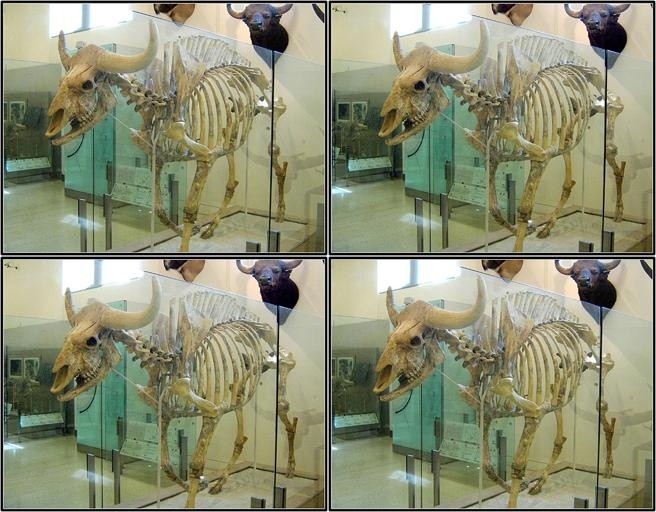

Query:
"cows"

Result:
[[563, 3, 633, 69], [233, 258, 302, 327], [552, 257, 623, 324], [224, 1, 295, 70]]
